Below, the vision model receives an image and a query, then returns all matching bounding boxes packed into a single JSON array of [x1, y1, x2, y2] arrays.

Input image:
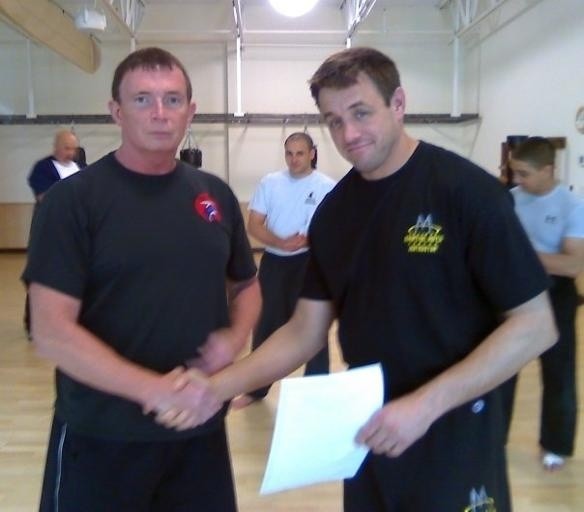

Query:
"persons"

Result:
[[21, 46, 265, 511], [500, 136, 584, 469], [230, 131, 339, 412], [22, 129, 88, 341], [140, 45, 563, 512]]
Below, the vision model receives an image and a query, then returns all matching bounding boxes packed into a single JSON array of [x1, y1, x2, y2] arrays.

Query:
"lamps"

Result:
[[75, 0, 107, 31]]
